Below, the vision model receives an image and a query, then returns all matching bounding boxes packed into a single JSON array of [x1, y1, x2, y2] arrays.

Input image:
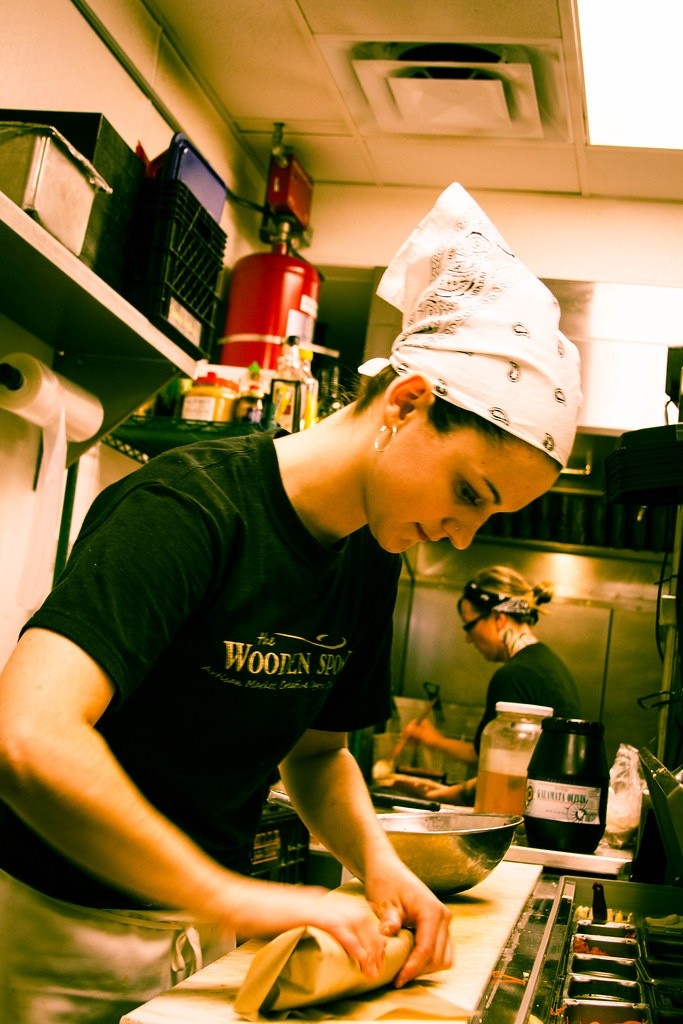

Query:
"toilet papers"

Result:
[[0, 351, 104, 489]]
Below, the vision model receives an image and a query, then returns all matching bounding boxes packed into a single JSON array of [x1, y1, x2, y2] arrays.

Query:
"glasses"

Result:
[[461, 615, 487, 635]]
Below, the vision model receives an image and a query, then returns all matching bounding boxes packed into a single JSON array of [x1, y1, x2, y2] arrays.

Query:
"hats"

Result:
[[353, 177, 585, 471]]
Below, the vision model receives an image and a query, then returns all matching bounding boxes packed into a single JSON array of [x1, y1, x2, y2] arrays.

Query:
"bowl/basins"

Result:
[[377, 811, 525, 897]]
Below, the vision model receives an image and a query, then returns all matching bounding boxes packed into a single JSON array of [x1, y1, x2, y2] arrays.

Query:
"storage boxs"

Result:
[[0, 106, 227, 359]]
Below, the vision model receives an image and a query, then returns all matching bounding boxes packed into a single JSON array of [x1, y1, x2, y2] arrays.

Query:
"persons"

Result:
[[386, 562, 582, 845], [1, 176, 585, 1024]]
